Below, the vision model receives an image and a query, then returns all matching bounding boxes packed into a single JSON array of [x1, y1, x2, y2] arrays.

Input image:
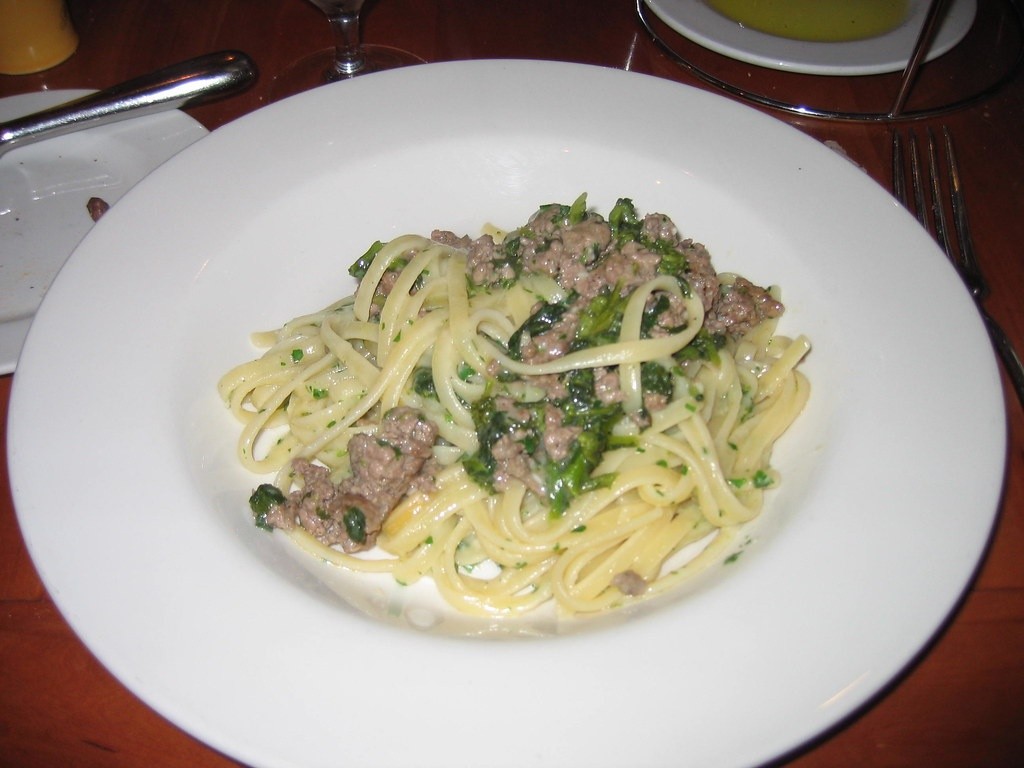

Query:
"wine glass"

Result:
[[266, 1, 427, 102]]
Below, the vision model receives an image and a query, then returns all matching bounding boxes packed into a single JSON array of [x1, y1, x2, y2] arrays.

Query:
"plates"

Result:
[[4, 59, 1006, 768], [632, 0, 1023, 123], [644, 0, 984, 77], [0, 90, 211, 378]]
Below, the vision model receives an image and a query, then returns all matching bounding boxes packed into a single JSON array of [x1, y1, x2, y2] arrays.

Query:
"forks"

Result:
[[890, 128, 1024, 423]]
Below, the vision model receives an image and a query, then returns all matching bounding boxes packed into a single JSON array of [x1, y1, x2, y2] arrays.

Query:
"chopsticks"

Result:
[[889, 0, 961, 122]]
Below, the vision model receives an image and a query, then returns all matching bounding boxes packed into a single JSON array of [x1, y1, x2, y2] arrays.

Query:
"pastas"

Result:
[[217, 234, 814, 614]]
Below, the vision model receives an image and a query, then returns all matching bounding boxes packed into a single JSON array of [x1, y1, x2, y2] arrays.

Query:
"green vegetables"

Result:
[[249, 193, 774, 548]]
